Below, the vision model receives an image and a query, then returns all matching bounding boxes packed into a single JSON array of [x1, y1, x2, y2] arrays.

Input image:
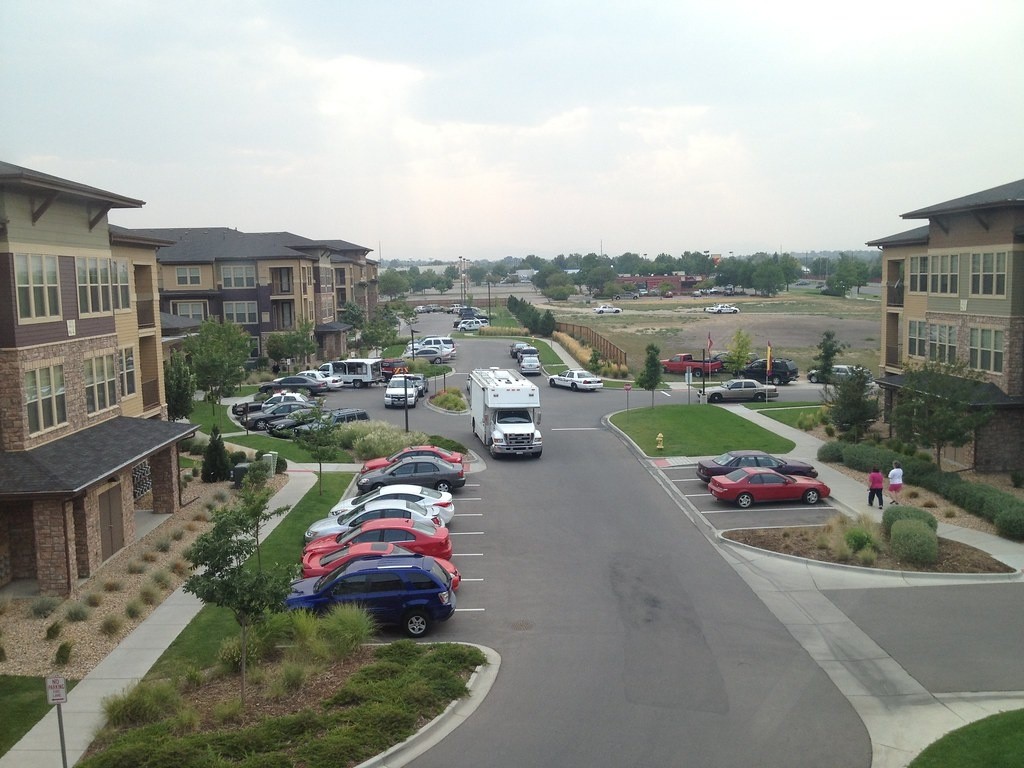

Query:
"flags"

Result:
[[707, 332, 713, 359], [767, 341, 772, 377]]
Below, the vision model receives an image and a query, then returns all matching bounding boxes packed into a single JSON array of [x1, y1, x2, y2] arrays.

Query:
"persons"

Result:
[[888, 460, 903, 505], [867, 466, 884, 509], [714, 302, 720, 307]]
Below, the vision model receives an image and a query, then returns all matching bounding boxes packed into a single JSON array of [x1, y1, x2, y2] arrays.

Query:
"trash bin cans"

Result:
[[234, 463, 250, 489]]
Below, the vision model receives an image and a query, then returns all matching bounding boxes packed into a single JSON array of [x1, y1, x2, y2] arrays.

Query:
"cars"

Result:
[[695, 449, 819, 485], [795, 280, 810, 286], [806, 364, 873, 386], [691, 285, 735, 298], [230, 300, 491, 448], [613, 290, 640, 301], [703, 351, 755, 375], [508, 341, 543, 377], [706, 466, 831, 509], [663, 291, 674, 298], [696, 378, 780, 404], [592, 304, 623, 315], [281, 442, 469, 640], [703, 303, 741, 315], [638, 288, 649, 297], [546, 368, 604, 393]]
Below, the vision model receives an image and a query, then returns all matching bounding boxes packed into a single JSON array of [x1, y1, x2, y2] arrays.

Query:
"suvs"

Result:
[[732, 355, 800, 386]]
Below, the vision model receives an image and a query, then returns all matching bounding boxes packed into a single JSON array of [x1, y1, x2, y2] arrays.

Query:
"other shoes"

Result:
[[896, 503, 898, 504], [890, 500, 895, 504], [879, 506, 882, 509], [868, 504, 872, 506]]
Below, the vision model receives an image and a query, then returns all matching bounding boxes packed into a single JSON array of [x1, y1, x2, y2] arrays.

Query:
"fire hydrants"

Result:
[[655, 432, 664, 450]]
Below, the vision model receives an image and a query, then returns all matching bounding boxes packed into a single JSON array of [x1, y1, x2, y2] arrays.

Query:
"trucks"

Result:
[[466, 366, 544, 461]]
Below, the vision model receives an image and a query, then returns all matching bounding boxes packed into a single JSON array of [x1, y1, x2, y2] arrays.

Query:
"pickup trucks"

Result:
[[658, 352, 722, 379]]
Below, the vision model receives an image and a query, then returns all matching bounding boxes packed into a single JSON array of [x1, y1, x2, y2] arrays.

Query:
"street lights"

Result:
[[459, 255, 464, 300], [463, 257, 471, 300]]
